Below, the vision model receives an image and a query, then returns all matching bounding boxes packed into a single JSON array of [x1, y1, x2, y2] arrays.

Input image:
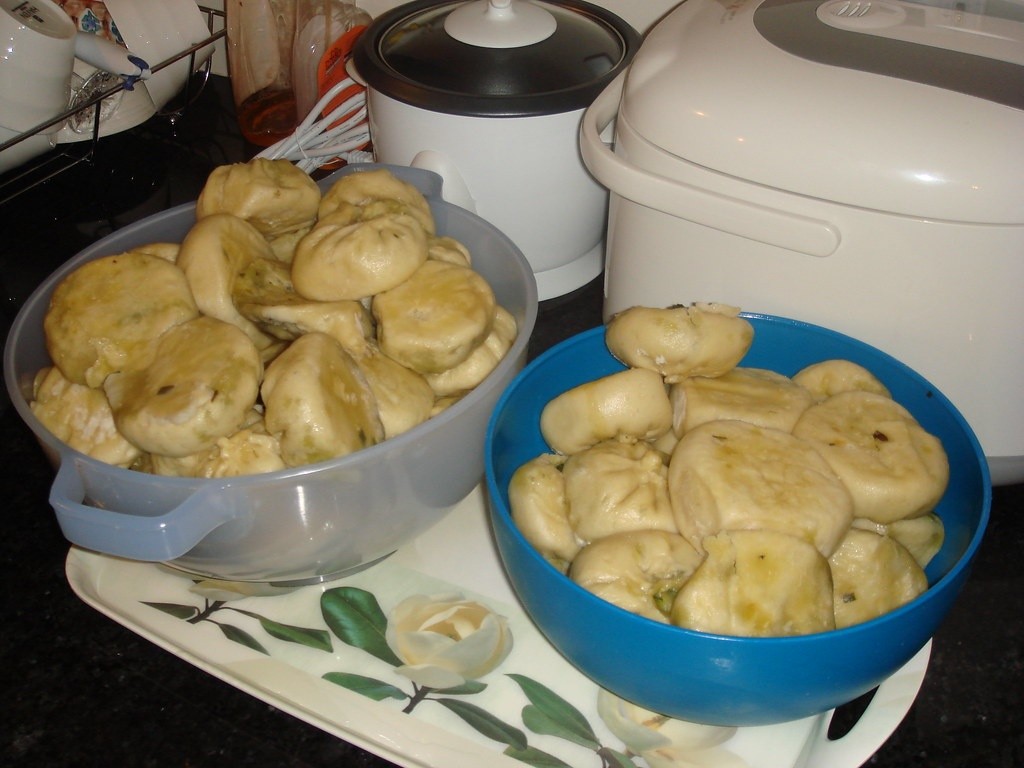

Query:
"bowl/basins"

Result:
[[103, 0, 216, 111], [483, 313, 993, 726], [0, 126, 57, 174], [0, 0, 75, 134], [7, 160, 538, 582]]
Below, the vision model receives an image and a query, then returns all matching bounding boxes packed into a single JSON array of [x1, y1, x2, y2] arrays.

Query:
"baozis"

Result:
[[510, 306, 948, 637], [31, 159, 514, 480]]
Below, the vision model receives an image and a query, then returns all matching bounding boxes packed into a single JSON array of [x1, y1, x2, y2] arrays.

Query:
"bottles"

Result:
[[292, 0, 373, 172], [227, 0, 295, 147]]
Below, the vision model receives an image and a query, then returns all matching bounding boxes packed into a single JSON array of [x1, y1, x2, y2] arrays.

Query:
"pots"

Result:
[[348, 0, 642, 298]]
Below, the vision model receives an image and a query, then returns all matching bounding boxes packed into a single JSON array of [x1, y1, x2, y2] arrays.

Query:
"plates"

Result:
[[57, 59, 156, 143]]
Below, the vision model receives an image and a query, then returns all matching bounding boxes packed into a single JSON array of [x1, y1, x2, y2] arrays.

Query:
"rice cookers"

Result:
[[579, 0, 1024, 492]]
